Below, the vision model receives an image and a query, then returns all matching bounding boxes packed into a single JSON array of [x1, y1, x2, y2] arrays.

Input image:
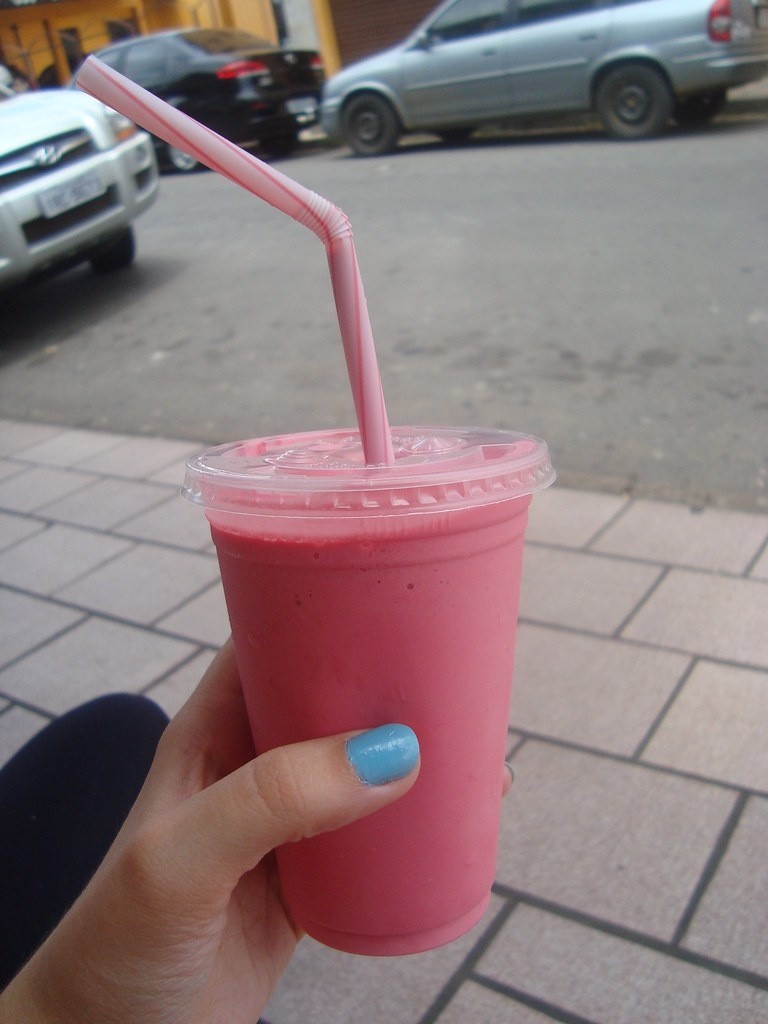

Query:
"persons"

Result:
[[0, 631, 517, 1024]]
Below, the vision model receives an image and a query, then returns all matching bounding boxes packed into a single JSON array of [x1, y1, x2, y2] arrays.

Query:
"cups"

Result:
[[177, 427, 555, 957]]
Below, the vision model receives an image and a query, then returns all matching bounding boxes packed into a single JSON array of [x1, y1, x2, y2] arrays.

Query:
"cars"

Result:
[[67, 26, 325, 172], [322, 0, 768, 158], [0, 84, 161, 290]]
